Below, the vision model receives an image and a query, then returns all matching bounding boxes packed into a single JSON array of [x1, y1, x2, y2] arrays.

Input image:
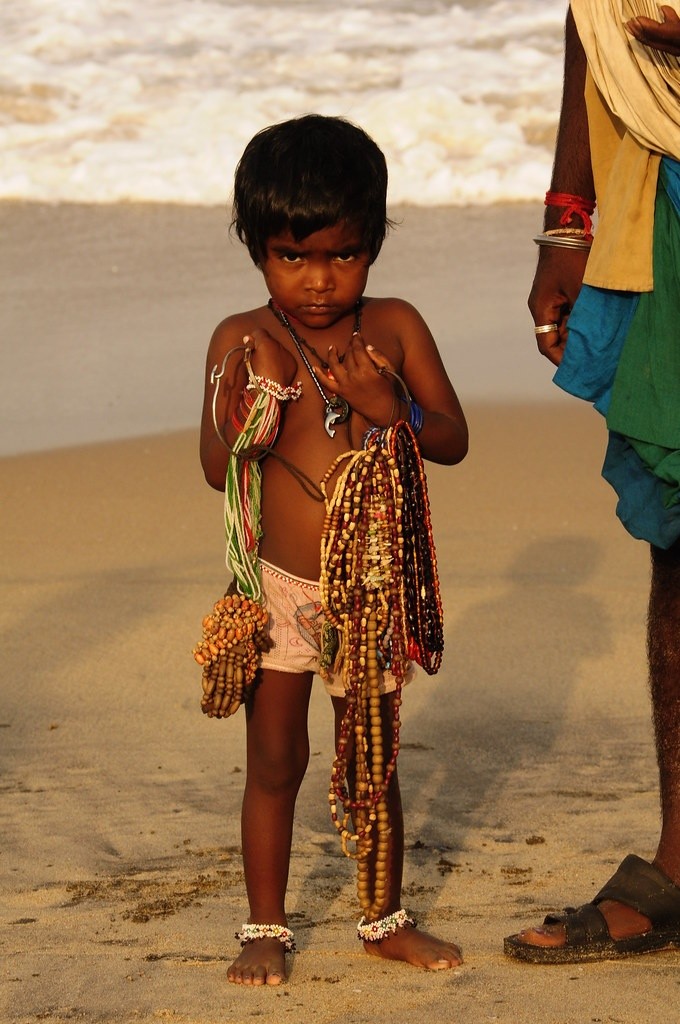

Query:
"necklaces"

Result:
[[268, 298, 364, 438], [317, 421, 444, 924], [222, 390, 282, 603]]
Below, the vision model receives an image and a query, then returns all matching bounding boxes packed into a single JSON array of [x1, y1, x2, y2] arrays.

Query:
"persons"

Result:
[[505, 0, 680, 963], [198, 114, 468, 984]]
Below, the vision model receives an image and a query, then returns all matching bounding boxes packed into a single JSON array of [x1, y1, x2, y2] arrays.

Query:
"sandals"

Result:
[[505, 852, 680, 964]]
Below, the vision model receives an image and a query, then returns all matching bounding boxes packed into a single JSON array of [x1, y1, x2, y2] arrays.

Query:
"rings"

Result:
[[535, 324, 557, 334]]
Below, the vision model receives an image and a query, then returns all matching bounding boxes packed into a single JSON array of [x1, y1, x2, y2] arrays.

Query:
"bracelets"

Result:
[[410, 404, 423, 434], [533, 229, 593, 250], [543, 191, 596, 234], [231, 375, 301, 431], [192, 594, 274, 719]]
